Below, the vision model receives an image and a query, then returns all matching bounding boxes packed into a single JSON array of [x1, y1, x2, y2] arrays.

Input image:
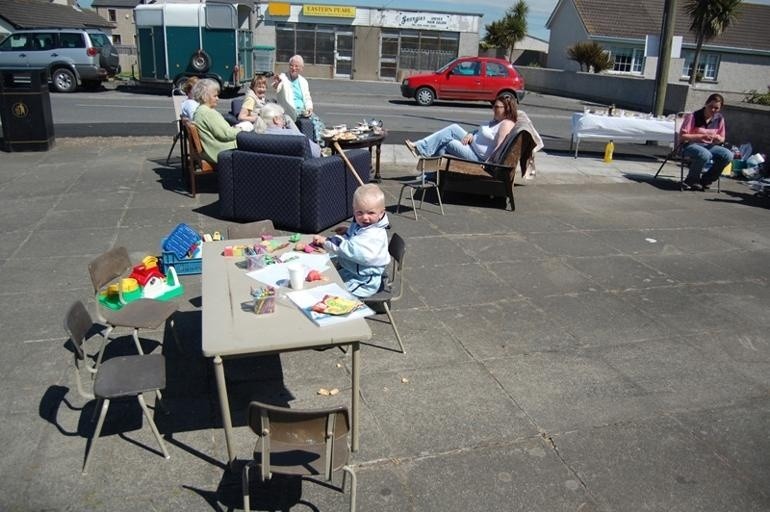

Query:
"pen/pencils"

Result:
[[251, 285, 276, 313]]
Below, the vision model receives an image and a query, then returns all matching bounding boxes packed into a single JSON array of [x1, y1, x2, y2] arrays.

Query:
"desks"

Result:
[[201, 233, 372, 479], [323, 127, 385, 183], [570, 112, 682, 159]]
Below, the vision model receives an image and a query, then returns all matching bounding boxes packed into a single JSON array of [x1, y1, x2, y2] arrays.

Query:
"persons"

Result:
[[675, 94, 733, 190], [406, 91, 517, 168], [181, 53, 330, 163], [311, 183, 391, 298]]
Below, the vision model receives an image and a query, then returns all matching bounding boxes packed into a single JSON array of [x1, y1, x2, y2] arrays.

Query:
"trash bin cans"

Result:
[[1, 86, 54, 152]]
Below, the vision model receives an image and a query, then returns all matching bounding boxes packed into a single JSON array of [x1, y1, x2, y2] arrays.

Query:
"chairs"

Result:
[[87, 246, 179, 382], [654, 112, 720, 194], [217, 132, 371, 233], [243, 401, 356, 512], [180, 115, 217, 198], [222, 98, 313, 141], [63, 302, 170, 473], [396, 156, 444, 221], [345, 234, 407, 356], [436, 110, 527, 209]]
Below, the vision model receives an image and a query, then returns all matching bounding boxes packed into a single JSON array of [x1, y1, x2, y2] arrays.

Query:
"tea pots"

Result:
[[363, 117, 384, 129]]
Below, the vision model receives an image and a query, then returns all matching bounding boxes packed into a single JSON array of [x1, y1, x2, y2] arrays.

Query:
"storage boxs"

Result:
[[730, 159, 747, 177], [162, 249, 202, 276]]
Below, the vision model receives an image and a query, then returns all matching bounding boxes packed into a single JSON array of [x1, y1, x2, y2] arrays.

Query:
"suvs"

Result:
[[0, 27, 121, 92]]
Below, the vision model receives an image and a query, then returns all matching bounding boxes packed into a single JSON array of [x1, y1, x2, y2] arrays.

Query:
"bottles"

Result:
[[734, 150, 742, 160], [341, 123, 347, 132], [604, 139, 616, 162], [335, 126, 342, 134], [746, 153, 766, 165]]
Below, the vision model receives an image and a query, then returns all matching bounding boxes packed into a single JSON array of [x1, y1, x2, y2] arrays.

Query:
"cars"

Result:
[[400, 56, 526, 106]]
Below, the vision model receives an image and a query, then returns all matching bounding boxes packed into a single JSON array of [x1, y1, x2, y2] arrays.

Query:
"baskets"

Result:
[[161, 237, 202, 275], [163, 223, 200, 258]]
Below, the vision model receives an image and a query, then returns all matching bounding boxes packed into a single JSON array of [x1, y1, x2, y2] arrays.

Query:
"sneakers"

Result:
[[416, 173, 427, 179], [404, 138, 419, 157], [742, 166, 761, 180], [683, 178, 707, 190]]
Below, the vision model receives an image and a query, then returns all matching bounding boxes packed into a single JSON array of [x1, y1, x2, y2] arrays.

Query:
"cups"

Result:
[[608, 106, 615, 116], [288, 261, 306, 291]]
